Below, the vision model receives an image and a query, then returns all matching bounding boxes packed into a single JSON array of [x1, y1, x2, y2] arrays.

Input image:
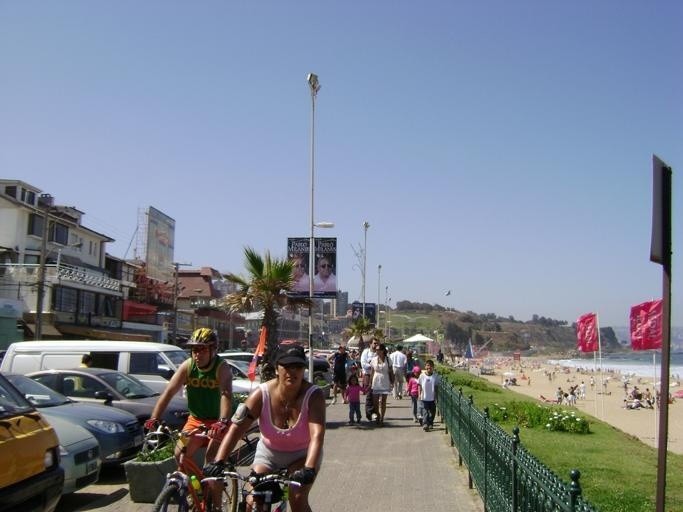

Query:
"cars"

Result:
[[306, 349, 344, 382], [216, 352, 274, 399], [1, 366, 193, 511]]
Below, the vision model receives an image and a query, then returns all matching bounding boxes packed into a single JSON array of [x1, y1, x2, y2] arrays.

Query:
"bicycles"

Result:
[[140, 418, 303, 511]]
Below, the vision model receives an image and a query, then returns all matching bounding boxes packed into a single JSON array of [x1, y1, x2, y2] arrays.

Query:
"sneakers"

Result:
[[422, 424, 428, 432], [341, 399, 346, 404], [428, 425, 432, 429], [331, 399, 336, 404]]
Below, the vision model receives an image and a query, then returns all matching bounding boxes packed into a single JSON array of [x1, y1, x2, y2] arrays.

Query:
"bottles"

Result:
[[189, 475, 204, 502]]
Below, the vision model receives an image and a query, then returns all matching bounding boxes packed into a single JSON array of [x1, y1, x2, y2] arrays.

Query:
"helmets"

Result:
[[184, 328, 217, 350]]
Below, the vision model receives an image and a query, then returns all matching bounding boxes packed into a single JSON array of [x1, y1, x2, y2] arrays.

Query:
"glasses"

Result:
[[294, 264, 304, 267], [280, 362, 305, 369], [320, 264, 332, 268], [373, 343, 377, 344], [191, 347, 209, 354]]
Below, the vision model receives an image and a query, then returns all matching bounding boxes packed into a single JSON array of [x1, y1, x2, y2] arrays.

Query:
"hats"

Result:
[[411, 366, 421, 373], [271, 344, 306, 369]]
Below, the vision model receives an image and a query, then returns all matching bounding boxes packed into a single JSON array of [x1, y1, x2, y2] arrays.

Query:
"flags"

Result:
[[575, 314, 598, 353], [629, 299, 664, 351]]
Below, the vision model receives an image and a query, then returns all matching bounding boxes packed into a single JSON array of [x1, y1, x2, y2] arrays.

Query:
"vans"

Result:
[[0, 338, 194, 397]]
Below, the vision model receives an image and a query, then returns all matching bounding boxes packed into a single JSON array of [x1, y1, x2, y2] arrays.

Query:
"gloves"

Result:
[[203, 461, 226, 477], [288, 468, 314, 484]]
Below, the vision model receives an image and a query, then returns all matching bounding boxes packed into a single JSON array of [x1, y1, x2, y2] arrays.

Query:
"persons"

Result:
[[314, 253, 336, 292], [287, 256, 309, 293], [327, 336, 444, 432], [202, 344, 327, 511], [455, 357, 675, 409], [144, 328, 233, 512]]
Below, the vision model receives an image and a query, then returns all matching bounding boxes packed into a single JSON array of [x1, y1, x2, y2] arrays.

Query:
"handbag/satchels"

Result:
[[386, 357, 395, 383]]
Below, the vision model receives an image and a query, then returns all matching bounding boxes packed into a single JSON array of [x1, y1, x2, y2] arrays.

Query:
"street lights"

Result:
[[376, 265, 382, 329], [362, 220, 370, 320], [384, 286, 392, 341], [306, 72, 321, 382]]
